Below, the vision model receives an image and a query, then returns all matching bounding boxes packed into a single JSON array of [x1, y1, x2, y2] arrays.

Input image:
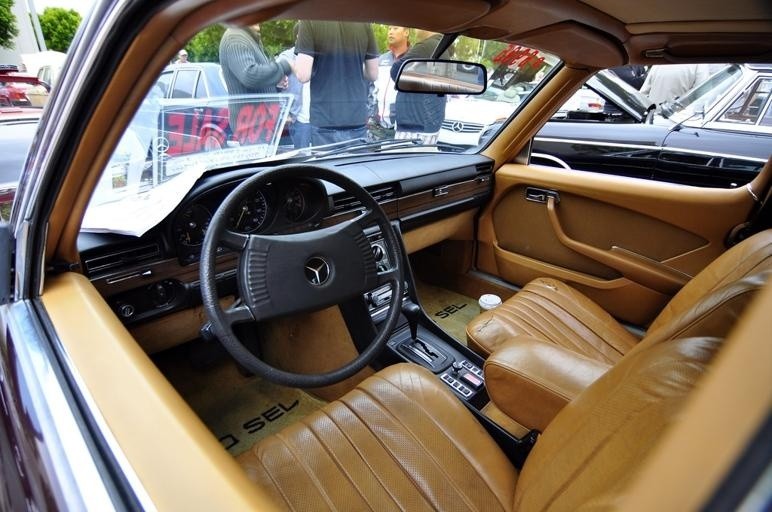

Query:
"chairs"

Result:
[[461, 225, 771, 355], [231, 274, 771, 512]]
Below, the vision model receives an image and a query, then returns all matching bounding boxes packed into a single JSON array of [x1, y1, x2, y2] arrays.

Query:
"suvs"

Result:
[[377, 52, 521, 149]]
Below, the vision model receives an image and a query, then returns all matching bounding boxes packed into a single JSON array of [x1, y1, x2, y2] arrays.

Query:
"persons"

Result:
[[214, 18, 455, 148], [602, 58, 711, 123], [177, 49, 190, 63]]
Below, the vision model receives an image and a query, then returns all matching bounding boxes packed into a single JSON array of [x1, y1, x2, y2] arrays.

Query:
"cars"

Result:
[[478, 63, 771, 187], [132, 63, 233, 155], [1, 64, 145, 217], [0, 0, 772, 510], [539, 88, 604, 118], [18, 50, 69, 87]]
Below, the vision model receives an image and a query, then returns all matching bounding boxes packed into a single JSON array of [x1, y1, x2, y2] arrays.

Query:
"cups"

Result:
[[474, 293, 505, 319]]
